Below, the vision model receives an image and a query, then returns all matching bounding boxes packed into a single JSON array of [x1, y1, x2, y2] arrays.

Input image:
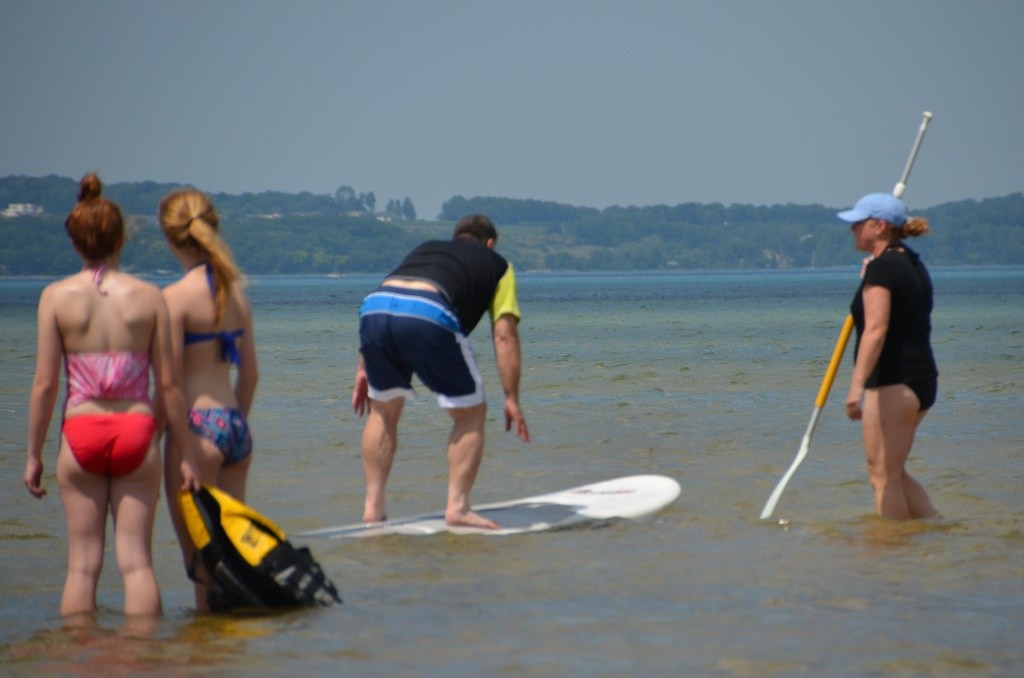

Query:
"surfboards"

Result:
[[297, 471, 683, 539]]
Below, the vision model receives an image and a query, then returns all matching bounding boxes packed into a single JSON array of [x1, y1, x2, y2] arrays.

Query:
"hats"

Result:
[[836, 192, 908, 228]]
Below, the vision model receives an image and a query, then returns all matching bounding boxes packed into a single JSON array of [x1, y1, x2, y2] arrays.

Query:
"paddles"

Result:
[[758, 108, 935, 524]]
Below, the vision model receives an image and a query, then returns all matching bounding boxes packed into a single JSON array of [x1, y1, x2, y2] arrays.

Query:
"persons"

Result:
[[350, 213, 531, 530], [156, 187, 258, 613], [23, 171, 201, 616], [835, 192, 945, 523]]
